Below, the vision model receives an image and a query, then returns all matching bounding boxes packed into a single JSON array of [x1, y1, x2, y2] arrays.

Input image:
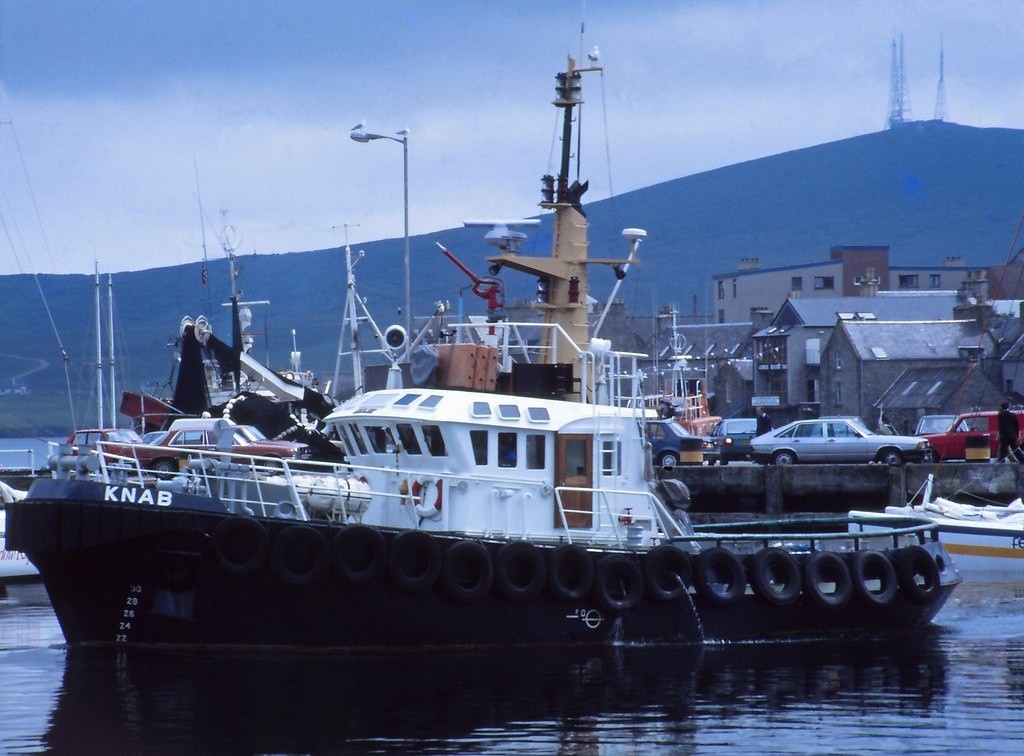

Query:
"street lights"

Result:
[[350, 120, 414, 364]]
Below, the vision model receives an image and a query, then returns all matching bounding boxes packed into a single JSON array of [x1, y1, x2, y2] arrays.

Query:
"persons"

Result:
[[498, 442, 516, 465], [998, 402, 1024, 463], [755, 406, 772, 437]]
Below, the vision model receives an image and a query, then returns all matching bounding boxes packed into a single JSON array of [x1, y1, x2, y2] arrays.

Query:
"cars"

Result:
[[750, 419, 931, 466], [62, 425, 312, 478], [914, 414, 970, 435], [709, 418, 775, 466], [809, 416, 866, 438], [636, 420, 720, 467]]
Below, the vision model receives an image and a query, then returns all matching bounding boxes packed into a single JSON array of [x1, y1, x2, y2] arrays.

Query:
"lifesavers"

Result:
[[640, 544, 694, 601], [743, 547, 803, 607], [591, 554, 644, 612], [382, 530, 444, 595], [267, 525, 332, 584], [438, 539, 497, 603], [802, 550, 853, 610], [546, 545, 596, 600], [849, 550, 899, 605], [326, 523, 388, 589], [412, 475, 443, 520], [490, 540, 548, 603], [210, 518, 271, 577], [694, 547, 749, 606], [896, 545, 942, 604]]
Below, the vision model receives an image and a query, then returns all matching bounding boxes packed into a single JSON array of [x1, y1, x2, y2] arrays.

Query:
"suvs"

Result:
[[916, 410, 1024, 462]]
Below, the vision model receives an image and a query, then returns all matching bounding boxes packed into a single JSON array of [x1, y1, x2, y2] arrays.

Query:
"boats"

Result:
[[0, 474, 41, 577], [6, 0, 963, 645]]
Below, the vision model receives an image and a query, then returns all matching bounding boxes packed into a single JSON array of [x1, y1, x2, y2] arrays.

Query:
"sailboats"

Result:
[[848, 215, 1024, 577]]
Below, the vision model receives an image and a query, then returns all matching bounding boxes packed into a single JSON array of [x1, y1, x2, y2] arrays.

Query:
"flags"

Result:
[[202, 260, 207, 284]]
